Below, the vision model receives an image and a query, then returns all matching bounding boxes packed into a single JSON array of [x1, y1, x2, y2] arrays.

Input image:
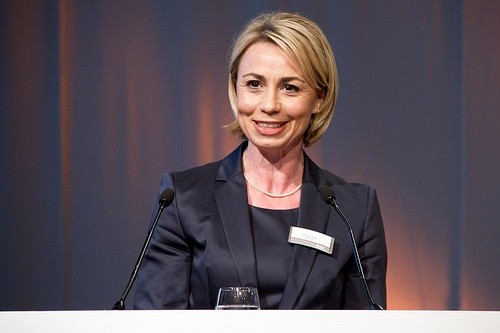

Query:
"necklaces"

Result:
[[241, 173, 301, 198]]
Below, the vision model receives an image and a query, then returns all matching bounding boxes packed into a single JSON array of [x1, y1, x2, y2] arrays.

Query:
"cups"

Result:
[[215, 287, 261, 310]]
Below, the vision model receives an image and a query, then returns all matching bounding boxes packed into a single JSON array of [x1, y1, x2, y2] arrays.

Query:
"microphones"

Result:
[[112, 187, 175, 309], [320, 187, 385, 309]]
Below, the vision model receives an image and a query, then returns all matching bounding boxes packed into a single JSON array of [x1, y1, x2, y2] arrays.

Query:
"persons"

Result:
[[134, 10, 389, 309]]
